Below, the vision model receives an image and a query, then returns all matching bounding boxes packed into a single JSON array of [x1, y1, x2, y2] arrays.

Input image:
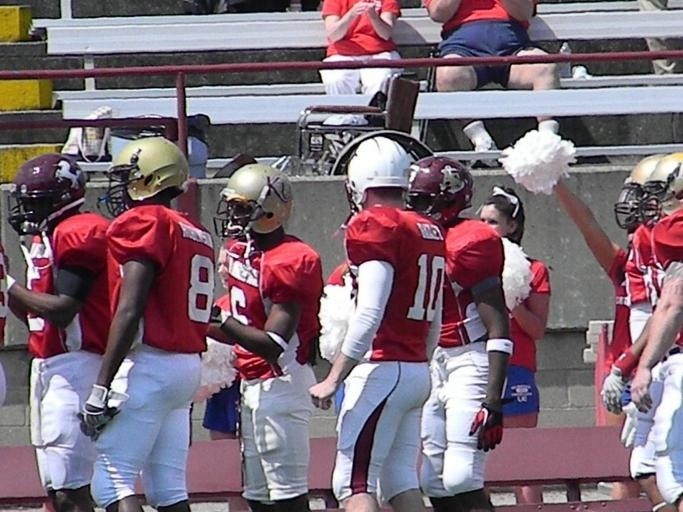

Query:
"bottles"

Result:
[[558, 41, 572, 79]]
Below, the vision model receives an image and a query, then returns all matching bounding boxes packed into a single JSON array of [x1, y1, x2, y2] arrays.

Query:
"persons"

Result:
[[318, 0, 403, 96], [420, 1, 564, 172], [636, 1, 677, 86], [79, 136, 215, 512], [311, 135, 550, 512], [207, 164, 325, 512], [0, 154, 110, 512], [555, 153, 683, 512]]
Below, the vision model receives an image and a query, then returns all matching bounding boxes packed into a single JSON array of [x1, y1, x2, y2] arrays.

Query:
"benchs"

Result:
[[24, 6, 682, 174]]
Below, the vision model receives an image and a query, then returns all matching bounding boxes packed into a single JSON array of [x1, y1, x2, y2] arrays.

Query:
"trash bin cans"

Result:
[[109, 113, 211, 178]]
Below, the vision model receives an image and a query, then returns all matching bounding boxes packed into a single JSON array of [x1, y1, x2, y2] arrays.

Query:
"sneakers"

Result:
[[470, 142, 500, 168]]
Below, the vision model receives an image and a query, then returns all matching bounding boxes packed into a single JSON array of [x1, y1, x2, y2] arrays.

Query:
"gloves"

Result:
[[599, 364, 629, 414], [469, 403, 503, 453]]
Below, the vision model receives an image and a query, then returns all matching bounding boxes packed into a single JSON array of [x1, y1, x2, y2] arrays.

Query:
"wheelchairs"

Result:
[[269, 71, 433, 177]]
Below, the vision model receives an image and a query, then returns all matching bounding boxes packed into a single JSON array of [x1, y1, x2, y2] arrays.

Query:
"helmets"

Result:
[[405, 157, 472, 226], [639, 153, 682, 228], [613, 152, 665, 231], [7, 152, 86, 235], [344, 137, 410, 213], [97, 136, 189, 220], [212, 163, 293, 242]]
